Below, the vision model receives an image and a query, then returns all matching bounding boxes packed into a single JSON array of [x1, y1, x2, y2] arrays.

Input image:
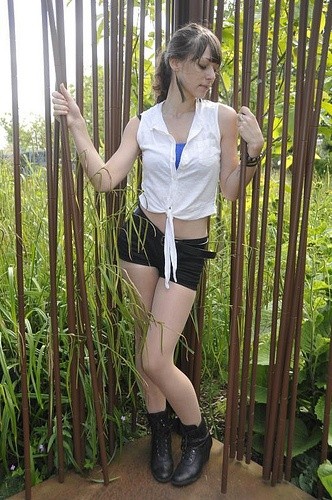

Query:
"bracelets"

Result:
[[238, 147, 261, 169]]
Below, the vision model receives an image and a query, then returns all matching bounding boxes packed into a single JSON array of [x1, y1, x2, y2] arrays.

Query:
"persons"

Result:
[[49, 20, 264, 488]]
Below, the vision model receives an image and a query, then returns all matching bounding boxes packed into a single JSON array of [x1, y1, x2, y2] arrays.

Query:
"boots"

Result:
[[146, 411, 175, 483], [172, 416, 212, 486]]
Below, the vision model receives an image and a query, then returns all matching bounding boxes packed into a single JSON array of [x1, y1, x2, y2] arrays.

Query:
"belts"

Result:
[[134, 208, 216, 260]]
